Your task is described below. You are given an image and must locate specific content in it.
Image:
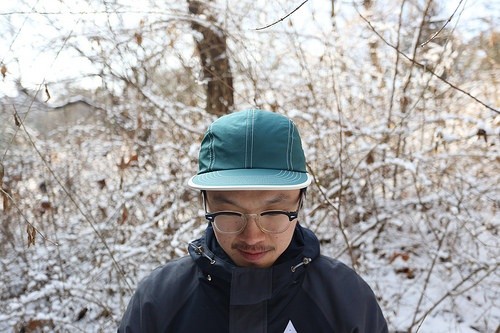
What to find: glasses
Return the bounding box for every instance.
[201,191,304,235]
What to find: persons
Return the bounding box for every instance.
[117,108,389,333]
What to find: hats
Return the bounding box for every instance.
[188,110,311,190]
